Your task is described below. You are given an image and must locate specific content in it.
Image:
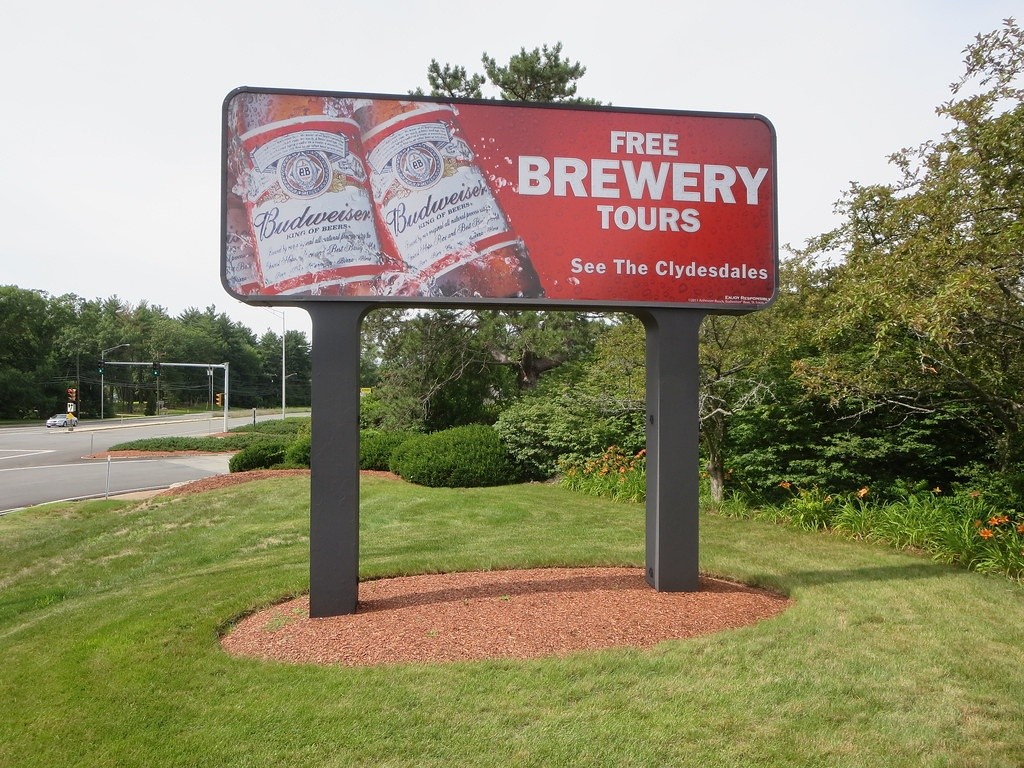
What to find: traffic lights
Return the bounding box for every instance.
[216,393,223,407]
[72,389,77,402]
[68,389,73,401]
[97,360,103,374]
[153,361,160,376]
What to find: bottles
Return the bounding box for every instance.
[351,98,544,299]
[232,92,385,296]
[226,173,262,297]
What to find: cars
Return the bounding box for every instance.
[45,414,78,427]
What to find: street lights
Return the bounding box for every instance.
[101,343,130,419]
[238,300,298,420]
